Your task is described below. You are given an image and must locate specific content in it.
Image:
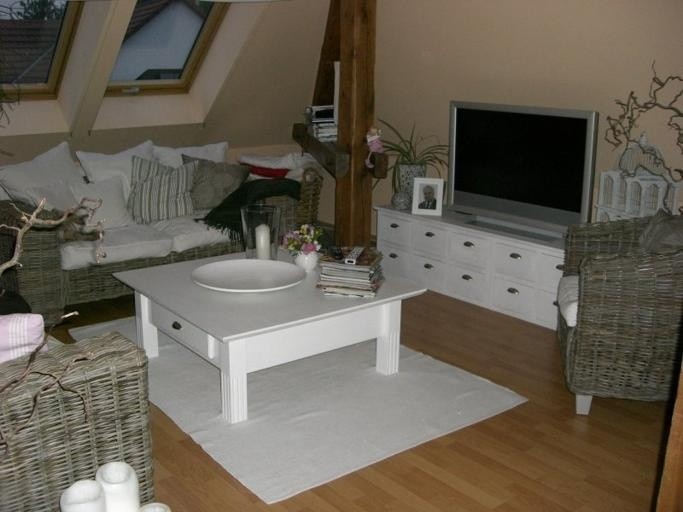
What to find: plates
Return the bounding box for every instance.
[191,258,308,294]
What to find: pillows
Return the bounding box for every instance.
[0,140,75,211]
[181,153,250,210]
[25,177,78,211]
[638,208,683,248]
[68,175,133,228]
[126,156,199,225]
[1,313,48,364]
[75,139,155,204]
[154,141,227,169]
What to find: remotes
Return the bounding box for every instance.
[329,244,343,260]
[345,246,366,265]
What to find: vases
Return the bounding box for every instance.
[391,192,411,209]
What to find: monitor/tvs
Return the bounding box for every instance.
[447,101,600,238]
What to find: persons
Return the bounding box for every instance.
[419,185,435,211]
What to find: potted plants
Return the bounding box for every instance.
[371,118,449,203]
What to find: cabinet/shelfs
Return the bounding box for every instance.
[373,204,565,332]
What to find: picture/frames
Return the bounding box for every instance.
[411,176,444,217]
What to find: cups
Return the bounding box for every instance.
[239,204,282,260]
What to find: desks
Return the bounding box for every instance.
[111,247,427,424]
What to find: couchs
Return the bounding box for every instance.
[0,143,324,327]
[556,216,683,415]
[0,290,155,511]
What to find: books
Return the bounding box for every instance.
[303,104,340,143]
[315,245,386,298]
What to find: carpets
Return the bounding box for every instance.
[68,315,528,506]
